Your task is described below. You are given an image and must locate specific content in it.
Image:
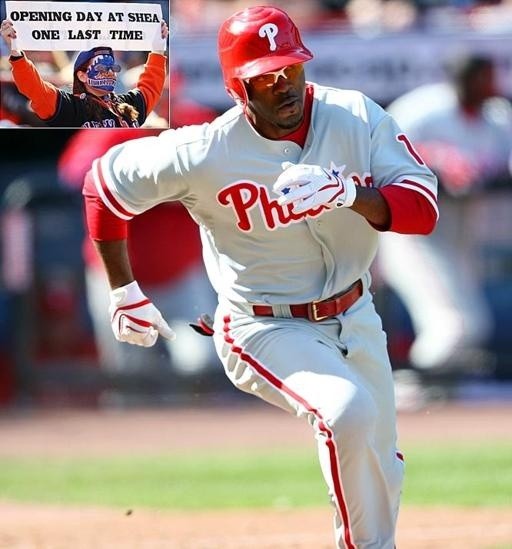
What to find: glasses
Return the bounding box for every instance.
[80,64,122,73]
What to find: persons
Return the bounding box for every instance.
[0,19,169,129]
[53,50,239,382]
[372,49,510,416]
[78,4,443,549]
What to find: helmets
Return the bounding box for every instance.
[217,14,313,109]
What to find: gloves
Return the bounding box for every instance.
[109,280,177,348]
[273,161,356,215]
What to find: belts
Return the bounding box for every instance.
[253,280,362,322]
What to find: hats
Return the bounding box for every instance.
[74,46,114,72]
